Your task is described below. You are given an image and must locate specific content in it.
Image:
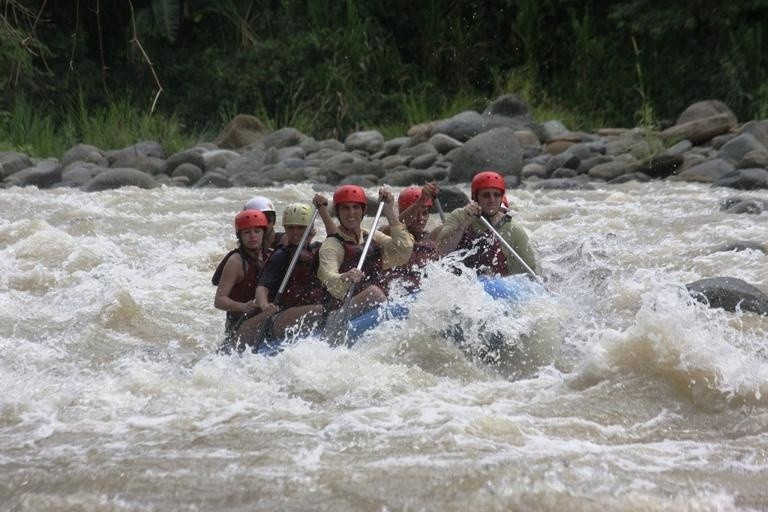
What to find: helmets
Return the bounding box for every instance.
[398,187,433,209]
[333,185,368,211]
[244,197,276,212]
[282,202,312,226]
[471,171,505,194]
[235,209,268,235]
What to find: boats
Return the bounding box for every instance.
[255,275,550,362]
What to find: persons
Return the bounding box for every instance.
[376,185,450,294]
[256,193,340,339]
[500,194,509,214]
[436,171,547,285]
[245,197,285,252]
[213,207,280,346]
[316,184,416,329]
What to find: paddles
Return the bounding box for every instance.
[249,203,328,351]
[321,199,385,346]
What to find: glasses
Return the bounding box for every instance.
[265,212,274,222]
[479,191,503,198]
